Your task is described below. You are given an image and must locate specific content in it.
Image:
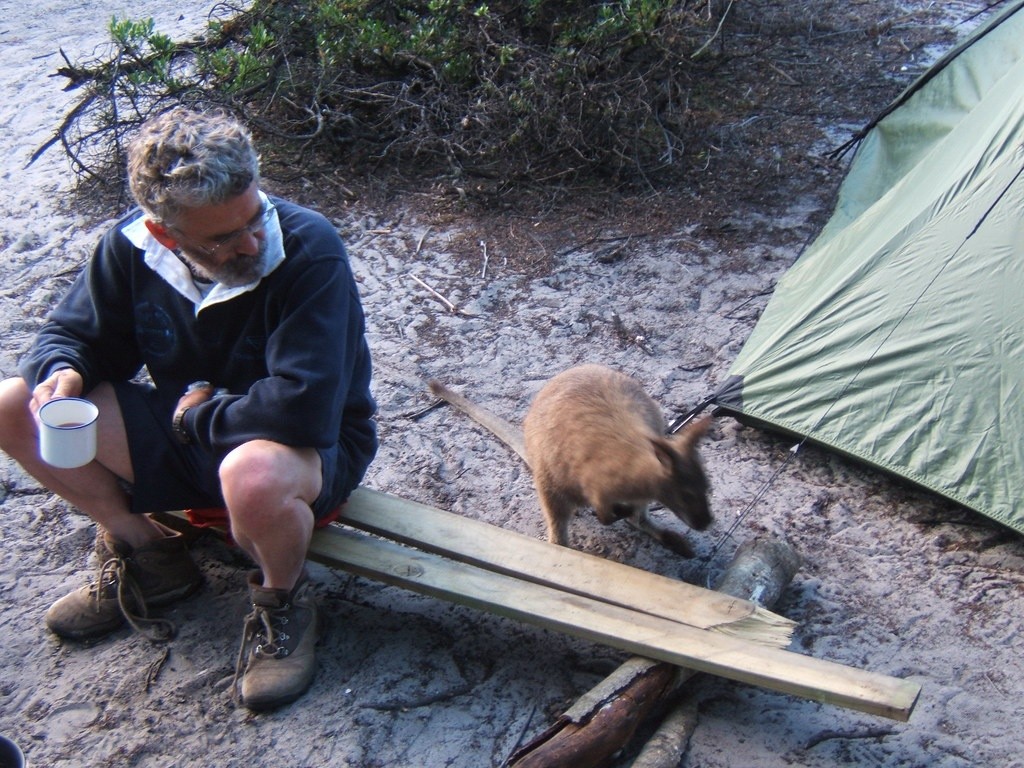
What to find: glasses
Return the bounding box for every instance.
[162,183,279,259]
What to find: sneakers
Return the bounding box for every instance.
[47,516,206,644]
[230,567,319,713]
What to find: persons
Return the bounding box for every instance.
[1,107,378,710]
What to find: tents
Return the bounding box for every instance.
[669,0,1024,533]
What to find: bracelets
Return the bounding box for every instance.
[174,407,194,446]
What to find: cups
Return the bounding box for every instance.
[38,397,99,469]
